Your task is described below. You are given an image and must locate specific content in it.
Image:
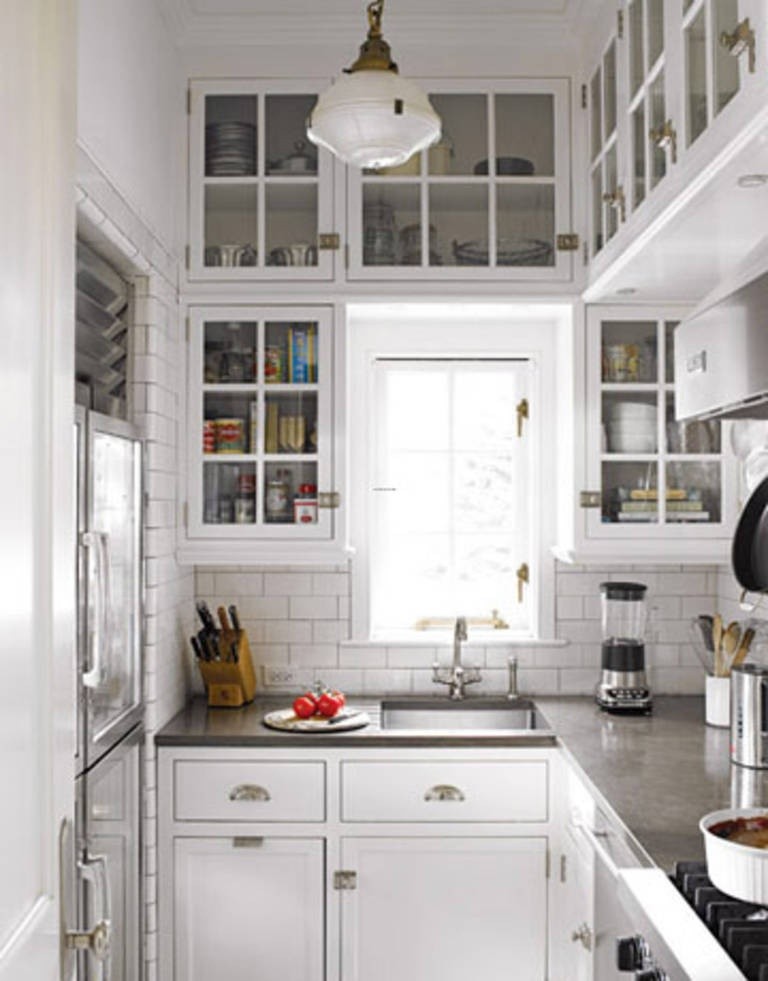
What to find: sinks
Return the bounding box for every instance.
[376,697,555,733]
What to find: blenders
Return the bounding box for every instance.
[594,583,654,713]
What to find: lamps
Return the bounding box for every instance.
[305,0,441,167]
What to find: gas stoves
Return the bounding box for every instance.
[616,859,768,980]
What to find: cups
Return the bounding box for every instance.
[600,343,639,380]
[363,203,396,263]
[731,663,768,769]
[402,224,443,265]
[704,674,730,730]
[206,120,256,175]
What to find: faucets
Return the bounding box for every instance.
[432,616,483,701]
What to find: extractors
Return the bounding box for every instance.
[673,257,768,424]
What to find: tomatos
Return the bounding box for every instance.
[294,687,346,718]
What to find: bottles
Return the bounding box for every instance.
[207,469,318,524]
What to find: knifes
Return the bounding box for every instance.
[188,600,241,663]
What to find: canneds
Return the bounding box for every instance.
[216,472,289,523]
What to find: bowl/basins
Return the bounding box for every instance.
[699,807,768,909]
[205,244,256,267]
[600,401,658,455]
[270,245,318,265]
[731,474,768,614]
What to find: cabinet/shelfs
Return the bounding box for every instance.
[170,0,768,566]
[159,744,595,981]
